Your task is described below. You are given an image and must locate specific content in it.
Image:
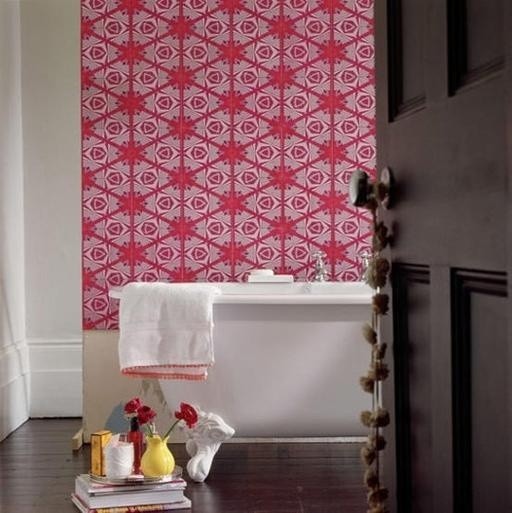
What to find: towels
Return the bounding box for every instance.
[118,283,213,381]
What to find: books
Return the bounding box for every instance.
[70,474,192,513]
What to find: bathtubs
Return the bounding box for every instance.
[105,281,379,438]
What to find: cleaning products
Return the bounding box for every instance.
[128,417,143,475]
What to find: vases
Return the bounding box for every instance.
[140,434,175,477]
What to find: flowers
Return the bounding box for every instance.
[124,396,158,437]
[162,400,198,442]
